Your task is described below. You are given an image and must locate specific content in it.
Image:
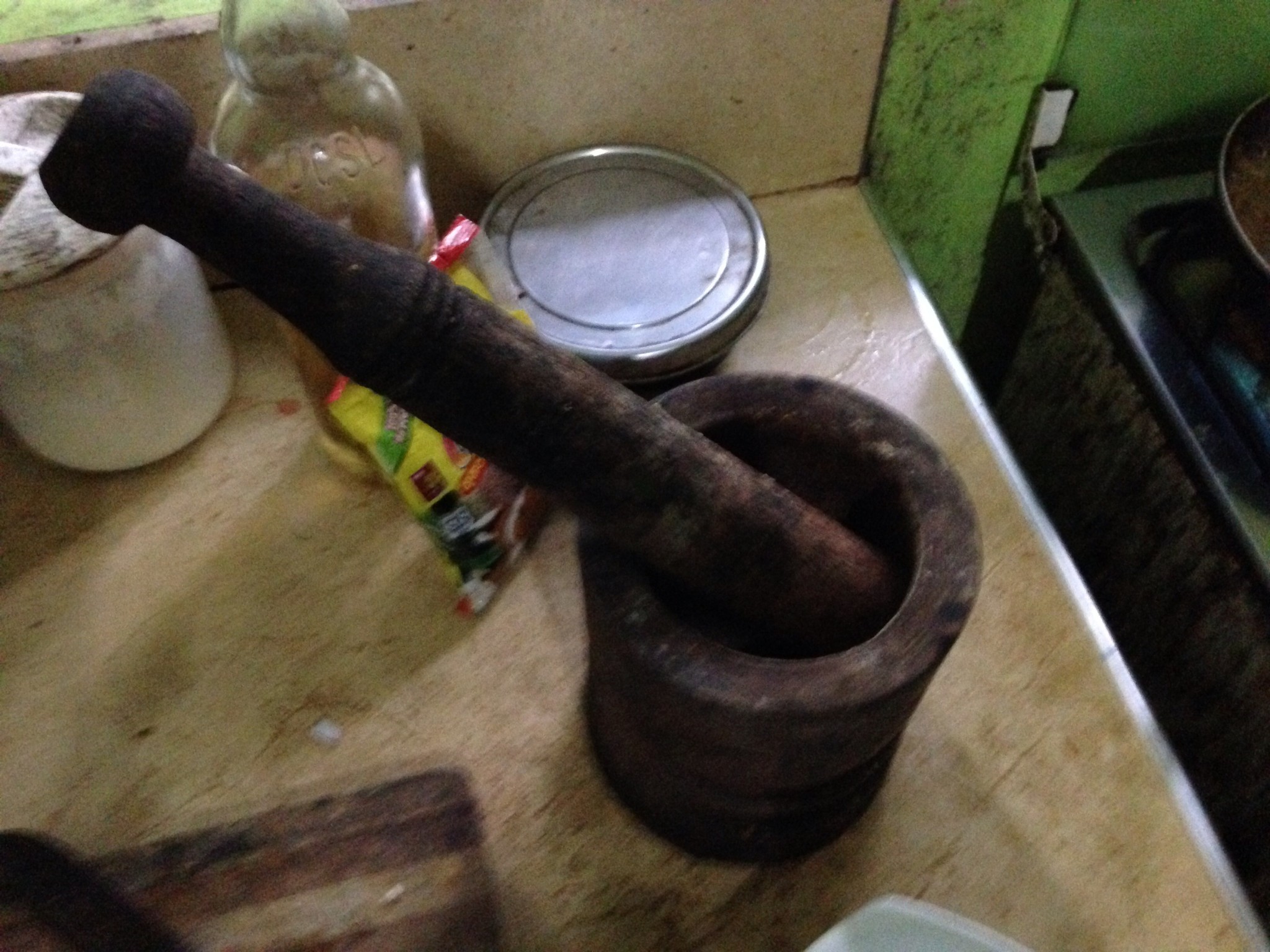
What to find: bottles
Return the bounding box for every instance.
[211,0,442,483]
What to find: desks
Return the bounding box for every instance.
[2,173,1268,952]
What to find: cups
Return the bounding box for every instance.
[585,367,984,872]
